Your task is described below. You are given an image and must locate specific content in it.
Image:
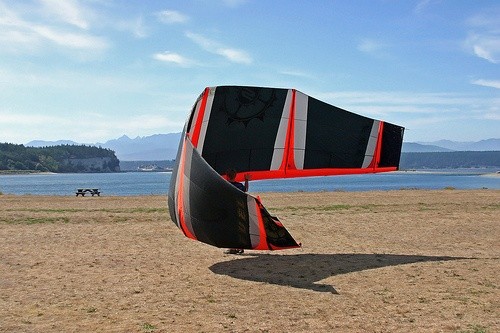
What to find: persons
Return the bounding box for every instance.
[224,169,251,255]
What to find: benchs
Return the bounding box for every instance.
[75,192,100,197]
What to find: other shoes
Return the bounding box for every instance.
[224,249,244,255]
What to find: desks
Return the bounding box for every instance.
[75,187,101,197]
